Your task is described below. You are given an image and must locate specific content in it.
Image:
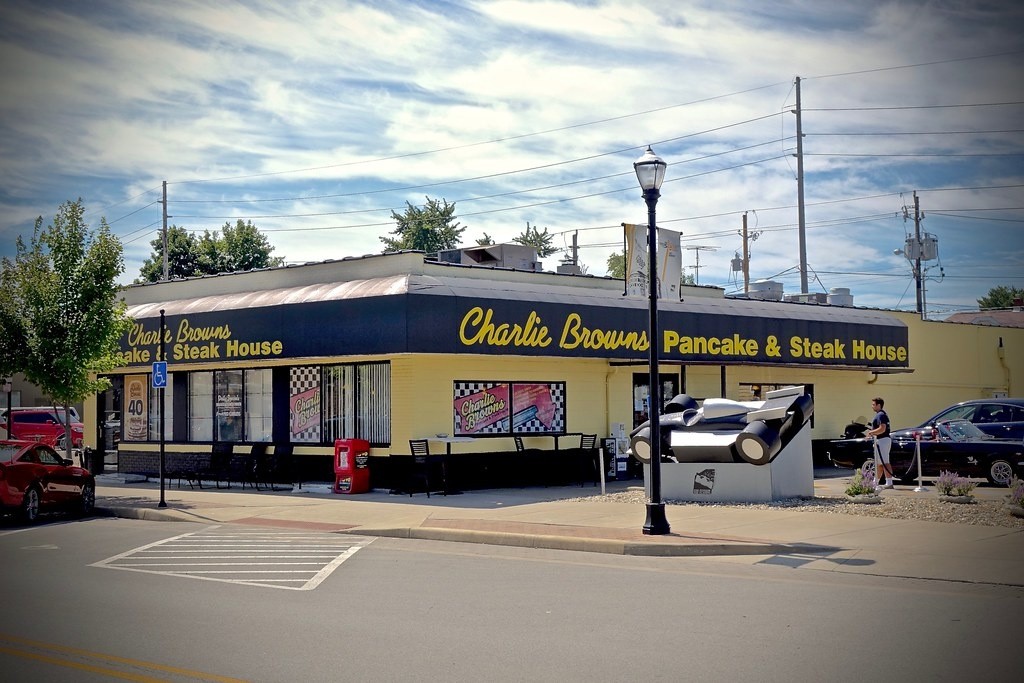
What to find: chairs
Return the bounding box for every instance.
[168,440,296,490]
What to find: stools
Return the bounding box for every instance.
[408,439,447,499]
[574,434,597,488]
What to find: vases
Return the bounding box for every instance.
[939,494,973,504]
[1009,506,1024,516]
[844,494,882,504]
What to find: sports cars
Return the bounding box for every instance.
[826,418,1024,488]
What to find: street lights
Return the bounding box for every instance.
[894,249,923,314]
[632,144,673,536]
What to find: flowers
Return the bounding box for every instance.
[933,469,974,496]
[1004,475,1024,506]
[846,466,877,495]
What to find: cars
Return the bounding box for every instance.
[0,439,96,526]
[909,397,1024,444]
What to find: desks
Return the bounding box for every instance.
[422,437,475,495]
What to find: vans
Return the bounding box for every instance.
[6,409,84,451]
[0,406,81,440]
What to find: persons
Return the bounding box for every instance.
[866,398,894,489]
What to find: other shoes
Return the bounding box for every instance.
[883,484,893,489]
